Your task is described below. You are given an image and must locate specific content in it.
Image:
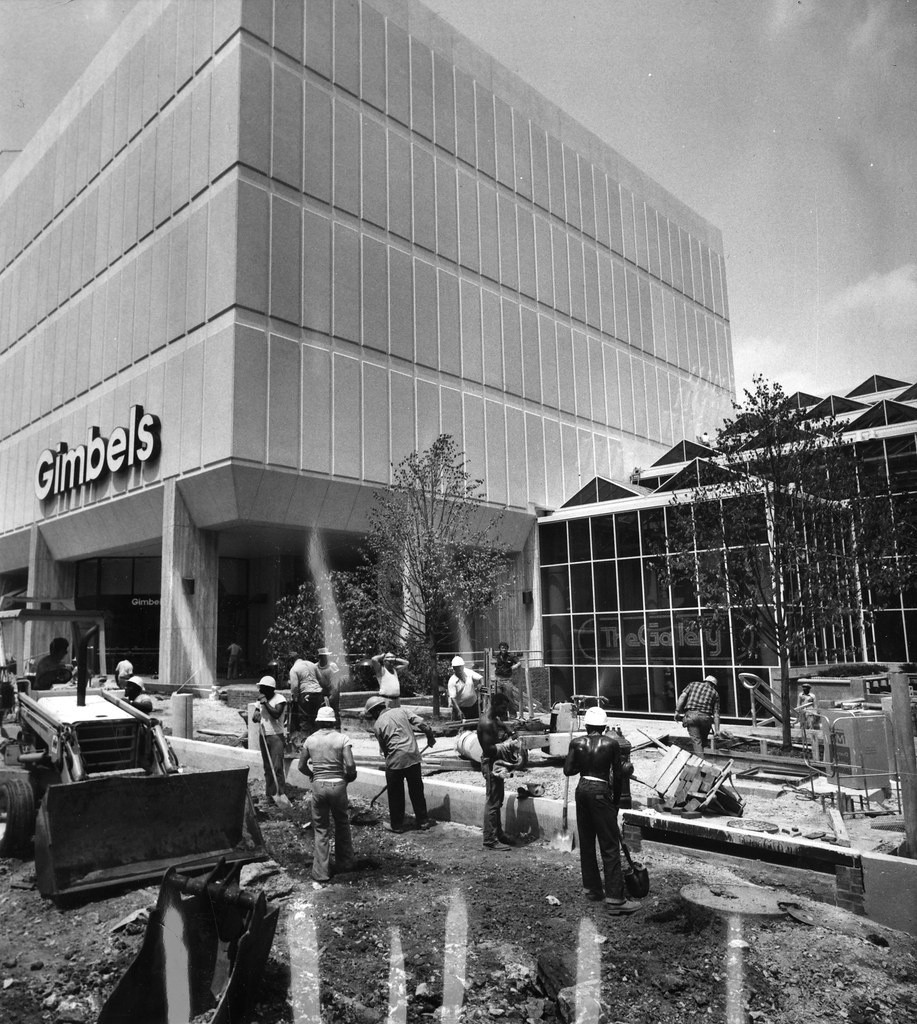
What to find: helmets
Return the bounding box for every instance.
[125,676,145,691]
[364,696,386,717]
[452,656,465,666]
[256,676,276,688]
[584,707,608,726]
[314,706,337,722]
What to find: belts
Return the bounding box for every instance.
[381,695,400,699]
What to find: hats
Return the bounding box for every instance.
[802,684,812,690]
[704,675,718,685]
[317,648,333,655]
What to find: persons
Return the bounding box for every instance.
[121,676,153,713]
[115,653,134,689]
[563,706,643,916]
[297,706,358,888]
[314,647,341,729]
[226,640,242,680]
[371,651,410,756]
[447,655,484,730]
[477,692,518,851]
[495,641,522,719]
[252,675,287,804]
[288,650,329,732]
[33,637,72,690]
[365,694,436,834]
[797,683,818,729]
[674,674,721,760]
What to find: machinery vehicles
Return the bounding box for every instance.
[0,594,272,900]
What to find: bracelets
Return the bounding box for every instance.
[675,709,680,712]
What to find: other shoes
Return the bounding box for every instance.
[498,836,516,846]
[483,840,511,851]
[607,901,642,914]
[383,820,403,833]
[585,889,605,900]
[350,857,368,872]
[418,818,436,829]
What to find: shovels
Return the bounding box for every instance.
[257,718,293,809]
[615,816,650,900]
[366,744,428,810]
[548,720,574,854]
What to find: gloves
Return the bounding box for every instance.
[257,693,268,704]
[251,711,260,723]
